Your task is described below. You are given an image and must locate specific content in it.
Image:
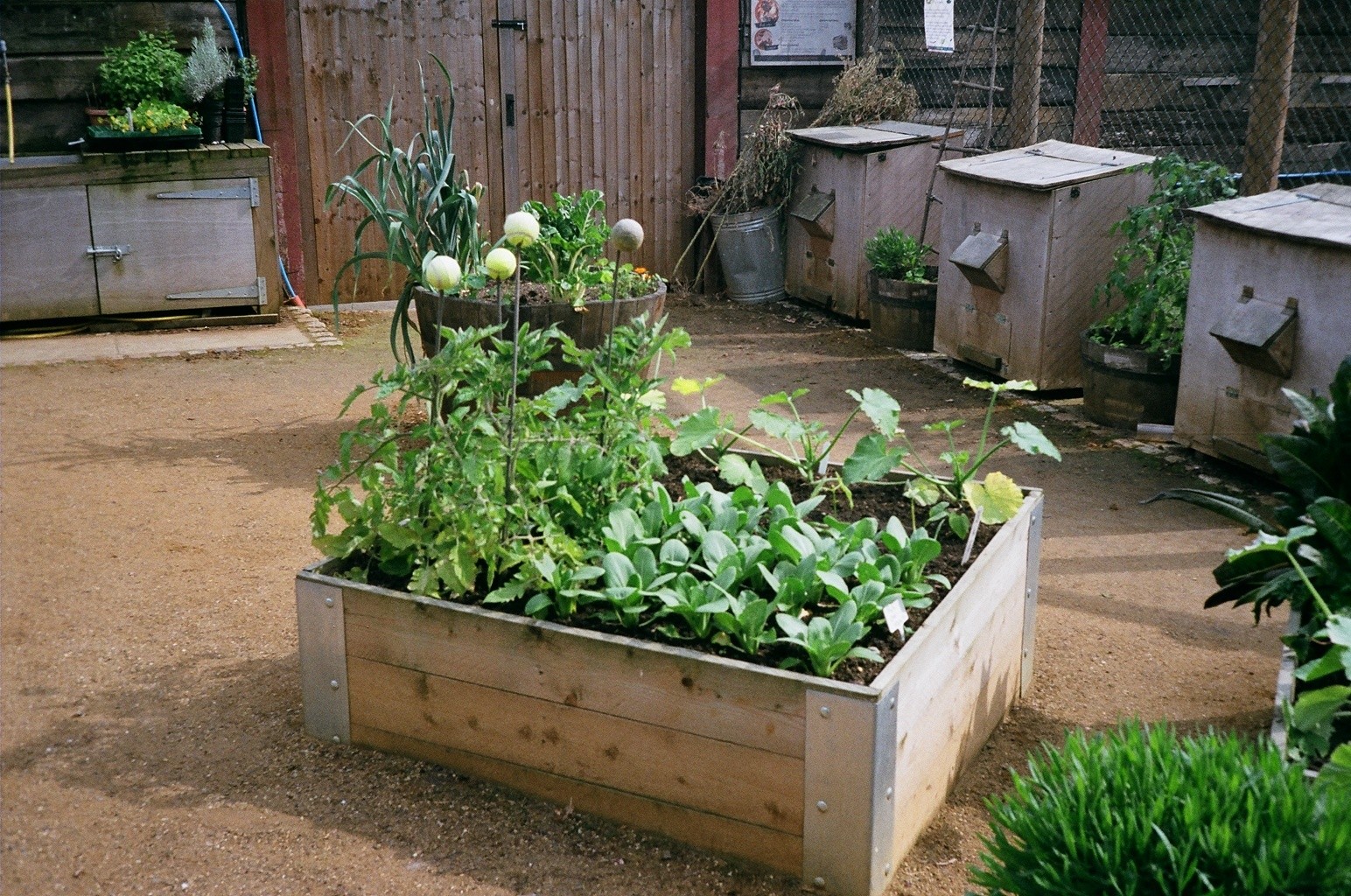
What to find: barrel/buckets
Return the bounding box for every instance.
[710,202,787,305]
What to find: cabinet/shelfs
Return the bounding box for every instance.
[0,138,282,336]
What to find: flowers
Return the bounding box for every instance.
[179,15,262,105]
[96,93,204,134]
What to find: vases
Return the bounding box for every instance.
[197,97,225,145]
[83,105,126,125]
[86,126,205,153]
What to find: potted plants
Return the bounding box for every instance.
[1078,152,1243,432]
[320,48,666,441]
[294,211,1064,896]
[861,222,938,353]
[1138,350,1351,782]
